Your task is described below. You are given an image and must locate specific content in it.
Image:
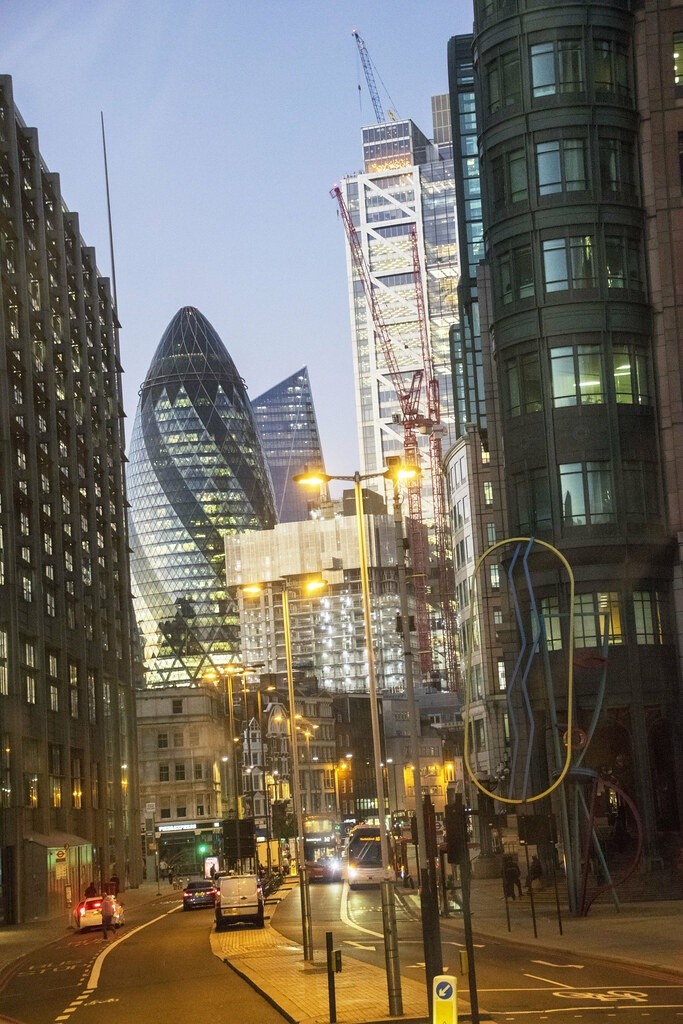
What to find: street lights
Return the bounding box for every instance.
[256,684,276,882]
[237,578,329,968]
[201,668,257,876]
[292,460,420,1015]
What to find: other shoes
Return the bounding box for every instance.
[113,930,116,935]
[103,937,108,939]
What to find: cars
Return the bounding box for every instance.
[182,879,217,911]
[73,894,126,934]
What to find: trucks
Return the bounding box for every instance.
[214,874,264,931]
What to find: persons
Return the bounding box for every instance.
[101,892,118,940]
[524,856,541,888]
[84,882,96,898]
[210,864,217,878]
[504,857,525,901]
[110,874,120,900]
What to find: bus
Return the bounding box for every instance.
[303,831,342,884]
[344,826,399,889]
